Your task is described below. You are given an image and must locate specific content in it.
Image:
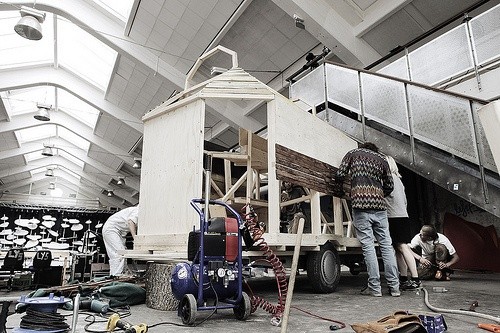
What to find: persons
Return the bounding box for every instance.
[407,225,460,281]
[102,207,141,277]
[337,142,423,297]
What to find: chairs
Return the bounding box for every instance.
[0,248,105,289]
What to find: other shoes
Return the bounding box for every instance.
[391,290,402,296]
[400,279,423,289]
[276,246,286,251]
[361,287,382,296]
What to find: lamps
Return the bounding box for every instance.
[34,103,142,197]
[14,0,47,41]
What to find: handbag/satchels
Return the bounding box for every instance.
[91,279,146,307]
[418,313,448,333]
[350,310,428,333]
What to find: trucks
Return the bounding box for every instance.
[120,45,409,294]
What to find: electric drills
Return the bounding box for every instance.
[107,313,150,333]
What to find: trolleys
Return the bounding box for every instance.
[170,199,252,326]
